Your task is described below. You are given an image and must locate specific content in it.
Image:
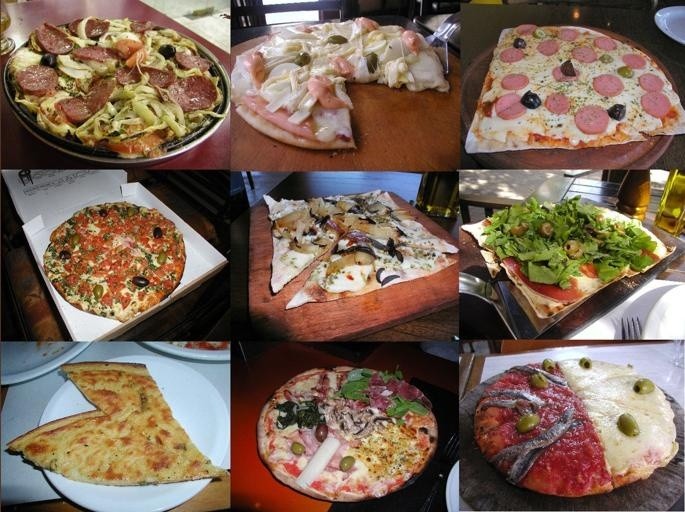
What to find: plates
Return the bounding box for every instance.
[1,341,231,512]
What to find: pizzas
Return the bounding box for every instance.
[260,189,458,310]
[465,22,685,154]
[43,203,187,323]
[256,366,439,503]
[5,361,226,487]
[231,15,450,150]
[463,199,676,320]
[473,357,680,498]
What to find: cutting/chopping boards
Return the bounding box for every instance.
[230,24,460,172]
[459,357,684,512]
[248,190,458,342]
[460,24,678,170]
